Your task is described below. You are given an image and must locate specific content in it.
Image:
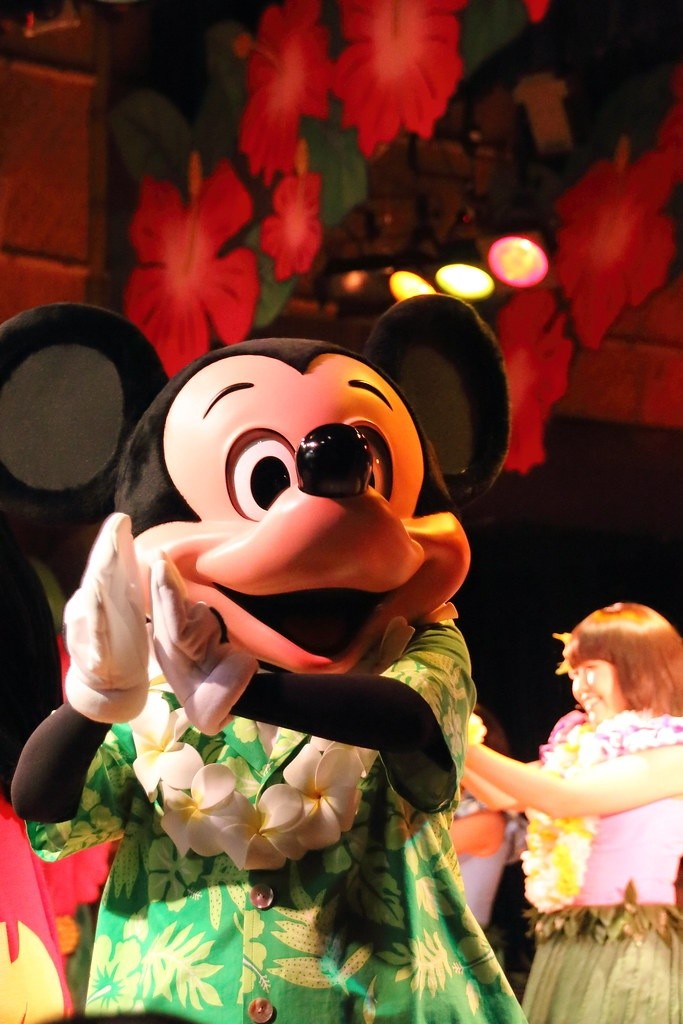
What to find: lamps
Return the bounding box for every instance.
[390,201,563,313]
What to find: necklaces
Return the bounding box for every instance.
[519,711,683,915]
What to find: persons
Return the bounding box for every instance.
[454,603,683,1024]
[455,707,515,931]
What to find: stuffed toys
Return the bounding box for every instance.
[0,287,526,1024]
